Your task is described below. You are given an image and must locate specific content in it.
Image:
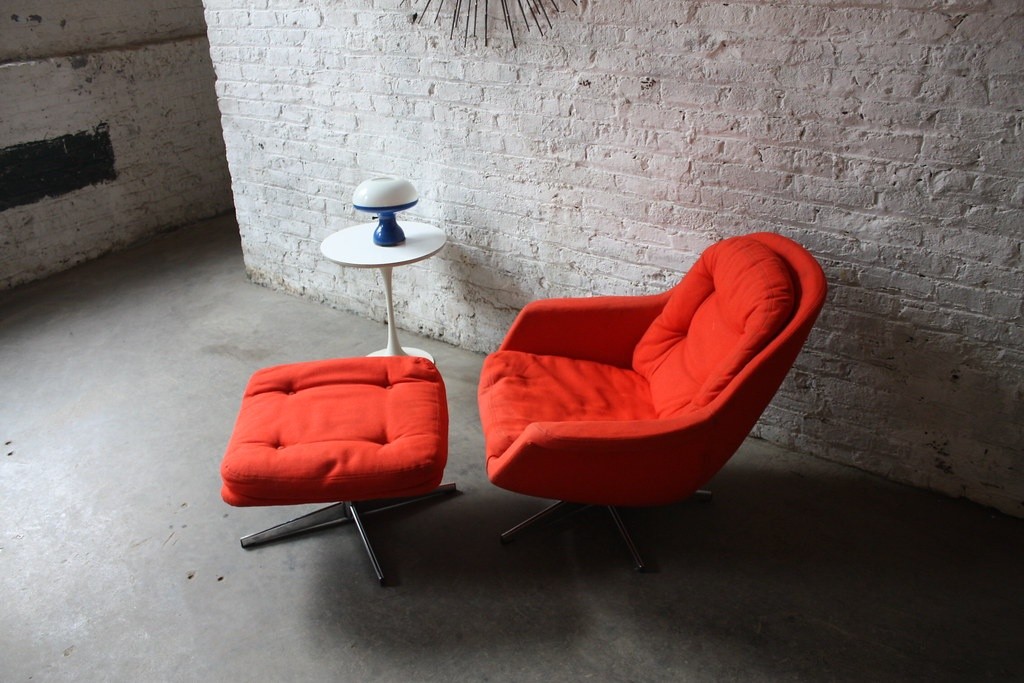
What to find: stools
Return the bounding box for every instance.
[221,357,456,586]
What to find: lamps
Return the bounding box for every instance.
[352,177,419,247]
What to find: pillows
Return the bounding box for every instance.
[632,235,796,418]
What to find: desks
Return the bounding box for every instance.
[321,220,448,365]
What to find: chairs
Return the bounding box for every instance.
[475,231,829,570]
